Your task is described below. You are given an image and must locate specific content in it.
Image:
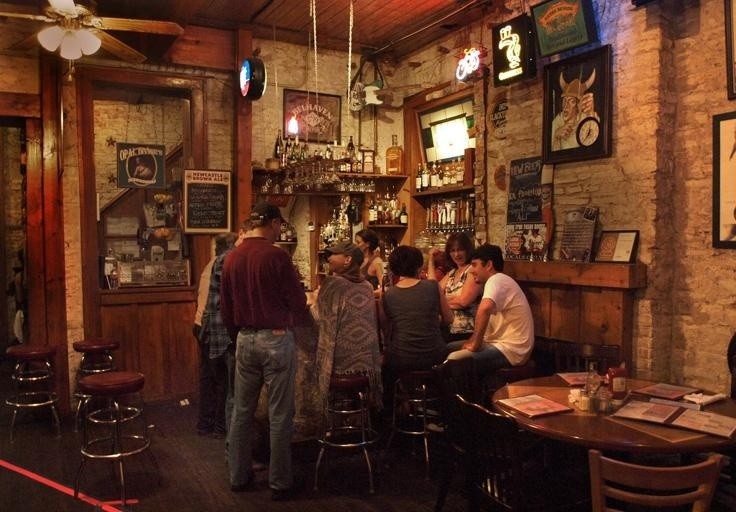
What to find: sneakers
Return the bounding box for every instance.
[426,422,449,433]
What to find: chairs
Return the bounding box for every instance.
[587,449,725,512]
[547,341,621,374]
[434,392,590,512]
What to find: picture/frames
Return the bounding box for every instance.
[592,230,639,263]
[712,112,736,249]
[541,45,612,165]
[530,0,598,59]
[103,214,141,238]
[116,142,166,189]
[283,88,342,145]
[723,0,736,101]
[104,238,143,260]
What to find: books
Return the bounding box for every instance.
[557,372,609,385]
[633,381,703,399]
[613,399,736,439]
[495,394,573,416]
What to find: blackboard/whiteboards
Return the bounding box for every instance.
[504,182,554,263]
[506,155,544,225]
[560,206,598,263]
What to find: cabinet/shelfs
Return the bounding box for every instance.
[252,166,408,292]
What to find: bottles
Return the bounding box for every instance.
[579,361,613,412]
[415,157,464,193]
[377,234,400,262]
[280,225,297,242]
[274,129,362,173]
[386,135,405,174]
[368,183,409,227]
[353,205,361,225]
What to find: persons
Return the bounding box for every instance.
[445,244,534,392]
[132,156,153,179]
[426,232,480,337]
[297,241,380,424]
[193,220,253,439]
[552,69,600,151]
[355,230,383,291]
[222,202,306,501]
[378,244,451,423]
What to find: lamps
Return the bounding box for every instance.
[33,20,103,60]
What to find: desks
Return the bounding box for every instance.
[491,372,735,456]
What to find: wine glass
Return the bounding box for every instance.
[262,163,377,195]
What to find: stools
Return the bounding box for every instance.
[72,338,120,434]
[312,374,380,497]
[382,370,438,481]
[490,366,532,381]
[5,345,62,442]
[74,371,158,508]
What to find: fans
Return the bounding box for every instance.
[0,0,184,64]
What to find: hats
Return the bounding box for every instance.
[325,242,365,265]
[248,201,289,224]
